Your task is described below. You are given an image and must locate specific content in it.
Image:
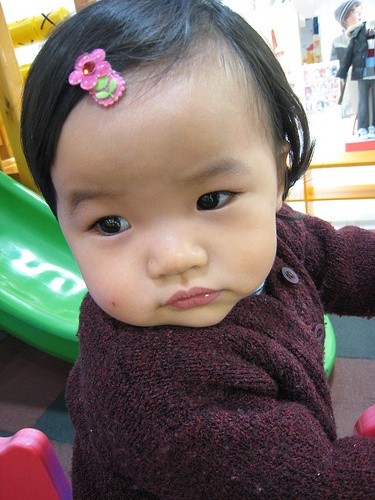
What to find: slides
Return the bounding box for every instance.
[1,172,336,382]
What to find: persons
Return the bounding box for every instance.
[334,19,375,136]
[329,1,364,63]
[21,0,375,499]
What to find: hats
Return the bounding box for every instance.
[334,1,359,27]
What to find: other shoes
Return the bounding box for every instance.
[358,126,375,139]
